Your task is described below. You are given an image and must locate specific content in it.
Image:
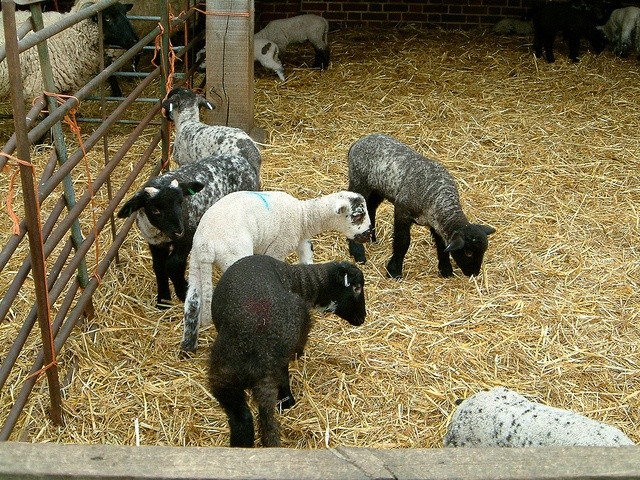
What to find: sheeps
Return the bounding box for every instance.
[117,154,261,322]
[597,6,640,61]
[162,88,262,190]
[443,385,636,448]
[179,190,376,360]
[491,18,536,35]
[1,0,141,148]
[71,1,208,98]
[254,14,331,76]
[207,254,368,447]
[347,133,498,281]
[521,5,609,63]
[196,40,287,82]
